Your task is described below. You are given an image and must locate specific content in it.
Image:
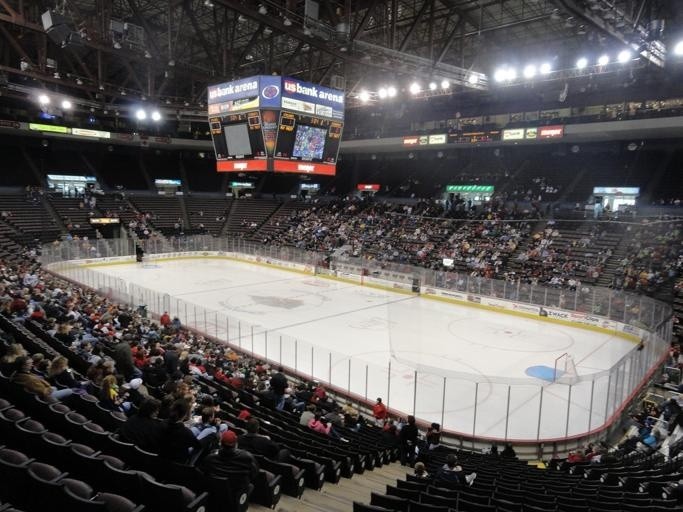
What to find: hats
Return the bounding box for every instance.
[130,378,143,389]
[222,431,236,443]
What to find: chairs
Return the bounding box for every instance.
[356,443,681,505]
[2,317,91,372]
[2,382,421,509]
